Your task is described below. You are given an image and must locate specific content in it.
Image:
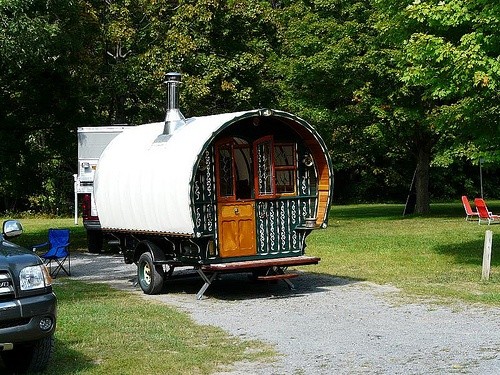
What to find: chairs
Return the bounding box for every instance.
[33,228,72,279]
[461,196,493,222]
[474,198,500,226]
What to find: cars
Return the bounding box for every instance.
[0,219,59,375]
